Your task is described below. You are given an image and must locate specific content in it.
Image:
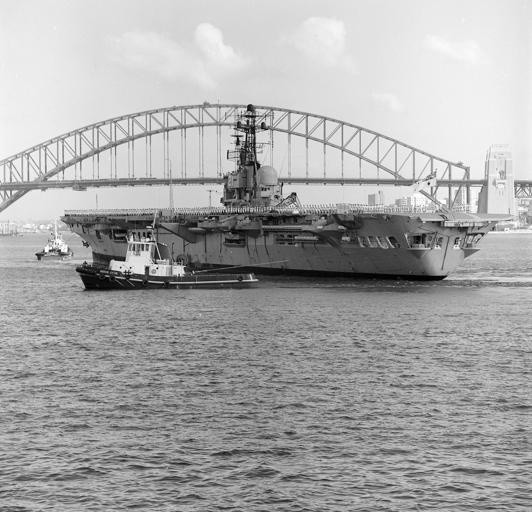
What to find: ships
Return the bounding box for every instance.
[61,103,513,281]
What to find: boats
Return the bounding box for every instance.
[75,211,289,290]
[35,222,74,262]
